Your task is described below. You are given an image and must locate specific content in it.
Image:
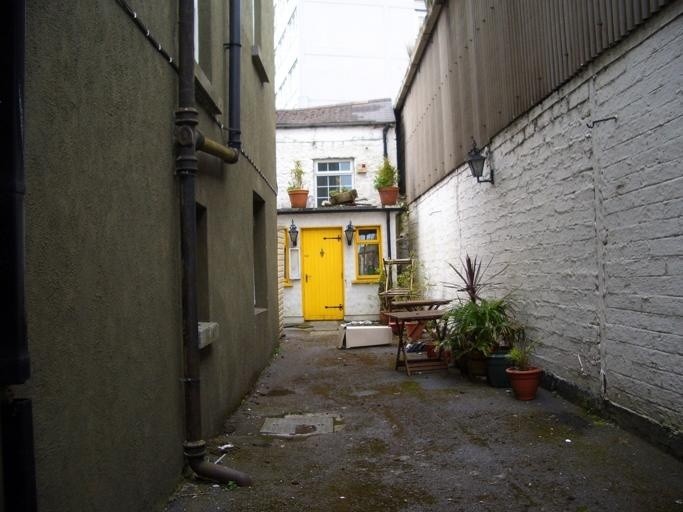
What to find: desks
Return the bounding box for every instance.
[385,294,453,378]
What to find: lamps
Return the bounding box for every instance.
[468,137,495,185]
[288,218,299,246]
[345,220,355,245]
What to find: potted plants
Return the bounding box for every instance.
[287,159,311,208]
[375,157,400,205]
[428,259,527,388]
[505,344,544,401]
